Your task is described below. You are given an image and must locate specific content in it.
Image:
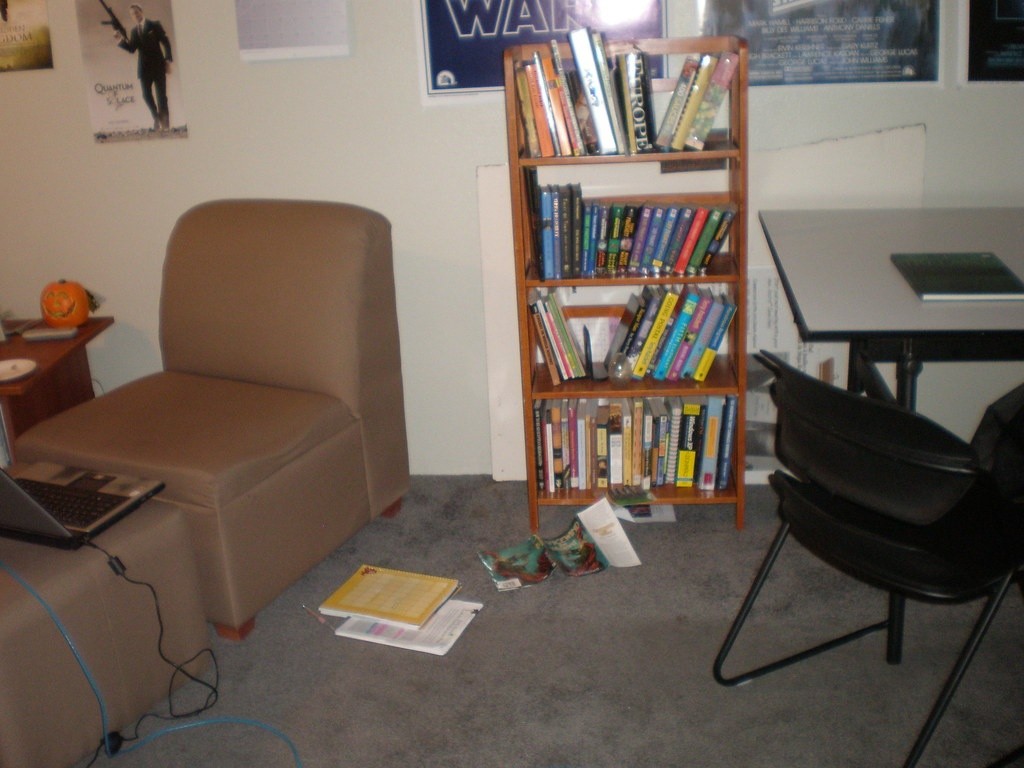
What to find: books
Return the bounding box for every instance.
[890,251,1024,302]
[319,563,484,658]
[531,393,738,498]
[527,287,587,386]
[514,26,740,159]
[603,284,738,383]
[522,165,737,283]
[477,498,643,593]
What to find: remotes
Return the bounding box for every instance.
[22,326,77,341]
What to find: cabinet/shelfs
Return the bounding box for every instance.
[502,35,749,538]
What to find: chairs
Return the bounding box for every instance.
[711,341,1024,768]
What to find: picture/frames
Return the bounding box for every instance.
[959,0,1024,86]
[413,0,677,105]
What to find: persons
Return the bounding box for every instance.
[112,4,173,132]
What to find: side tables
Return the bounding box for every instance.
[0,318,114,463]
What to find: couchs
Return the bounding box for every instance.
[0,497,212,768]
[15,196,411,643]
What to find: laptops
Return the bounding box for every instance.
[0,462,165,550]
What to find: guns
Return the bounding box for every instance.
[98,0,130,44]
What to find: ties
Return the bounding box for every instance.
[138,23,143,37]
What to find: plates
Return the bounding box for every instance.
[0,359,36,383]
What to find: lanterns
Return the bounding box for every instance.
[39,279,89,329]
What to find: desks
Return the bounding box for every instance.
[757,206,1024,693]
